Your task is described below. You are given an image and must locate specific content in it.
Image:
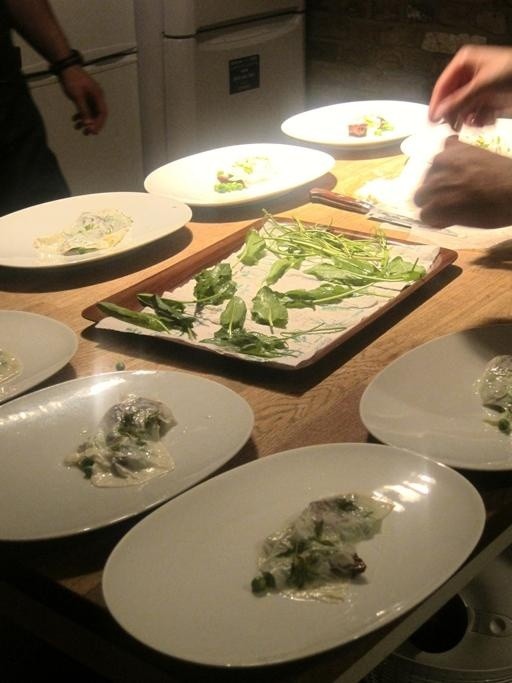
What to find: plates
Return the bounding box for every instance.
[0,191,194,273]
[357,321,511,474]
[279,99,445,151]
[142,142,335,209]
[399,117,512,160]
[79,213,460,376]
[99,440,489,667]
[1,307,257,544]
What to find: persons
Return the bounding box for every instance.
[0,0,108,216]
[413,43,512,230]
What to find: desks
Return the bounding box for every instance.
[0,112,512,683]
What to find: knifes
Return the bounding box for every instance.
[306,185,461,239]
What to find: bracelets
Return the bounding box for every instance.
[48,49,84,76]
